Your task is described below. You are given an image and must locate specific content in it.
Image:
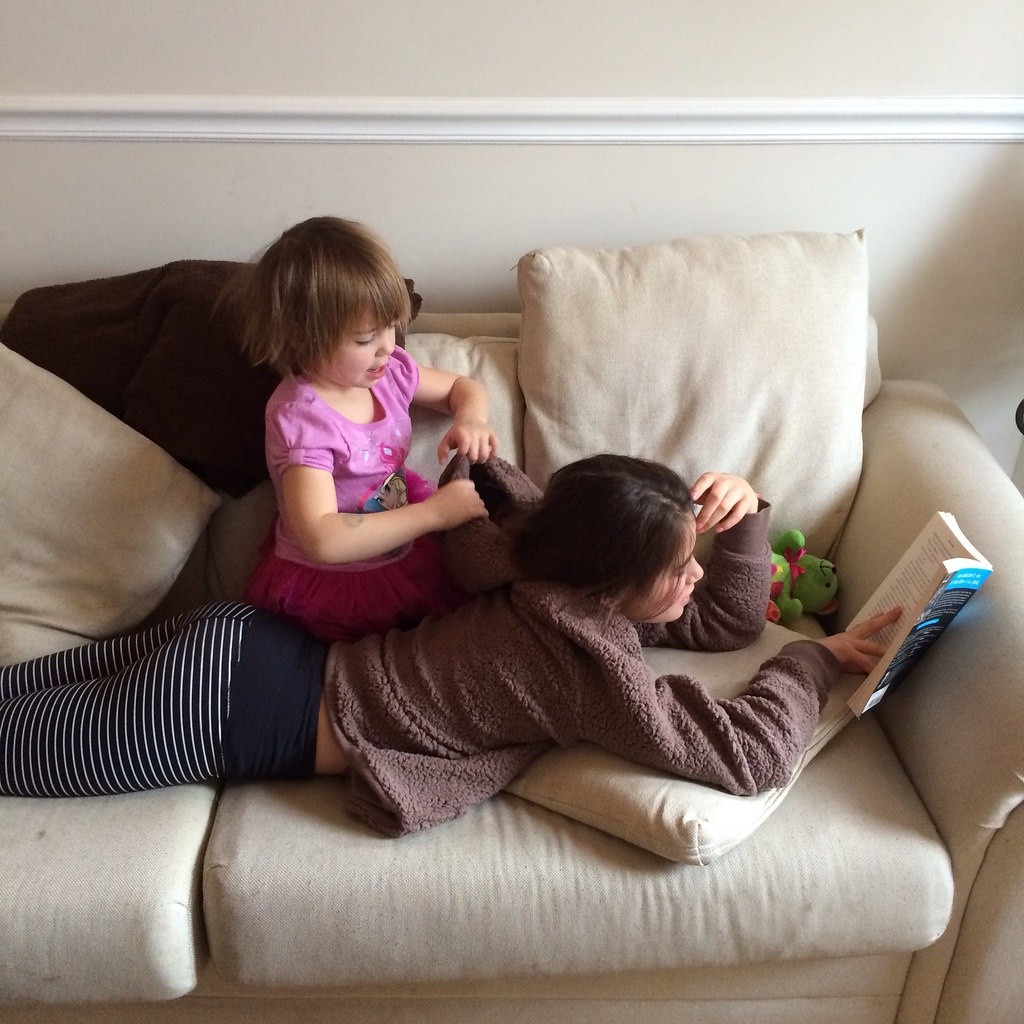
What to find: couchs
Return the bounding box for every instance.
[0,311,1023,1024]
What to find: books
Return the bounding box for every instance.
[845,510,992,723]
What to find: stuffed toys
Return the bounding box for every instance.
[767,526,842,624]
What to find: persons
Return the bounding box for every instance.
[243,216,502,644]
[2,448,904,841]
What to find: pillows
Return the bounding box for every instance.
[511,227,868,586]
[205,333,524,608]
[0,340,225,672]
[497,619,868,867]
